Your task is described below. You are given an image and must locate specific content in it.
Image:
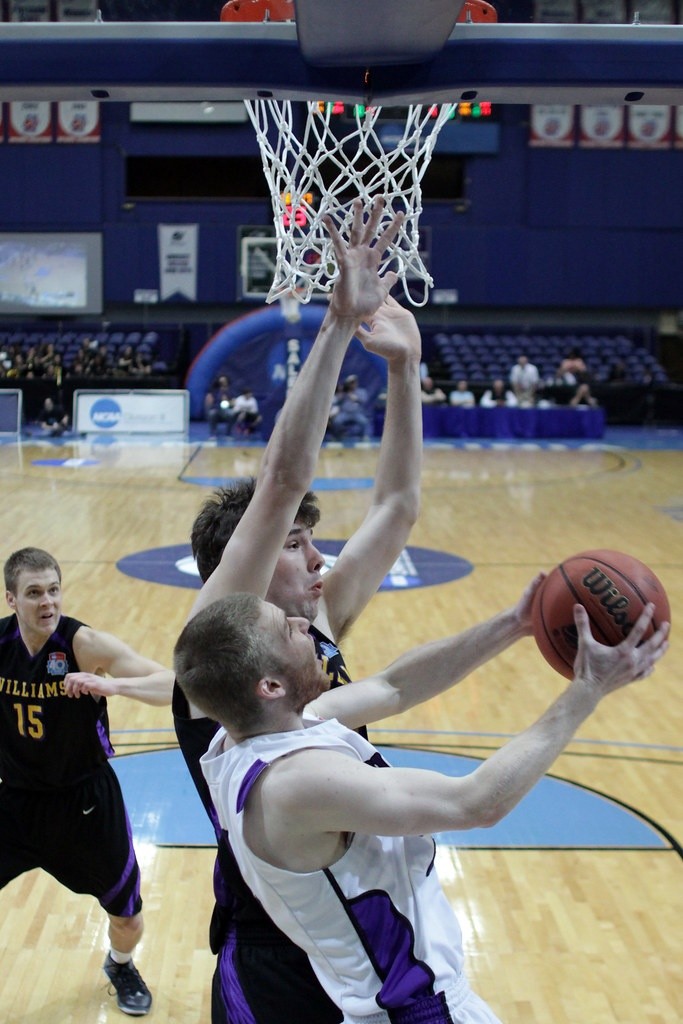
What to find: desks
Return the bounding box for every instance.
[372,407,607,441]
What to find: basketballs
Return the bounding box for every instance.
[532,547,672,684]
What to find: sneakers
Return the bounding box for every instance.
[103,950,152,1015]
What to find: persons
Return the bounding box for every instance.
[0,339,153,432]
[173,572,671,1024]
[420,352,598,410]
[0,547,175,1016]
[170,199,422,1023]
[201,374,372,443]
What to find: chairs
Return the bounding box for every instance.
[0,329,172,388]
[430,329,677,388]
